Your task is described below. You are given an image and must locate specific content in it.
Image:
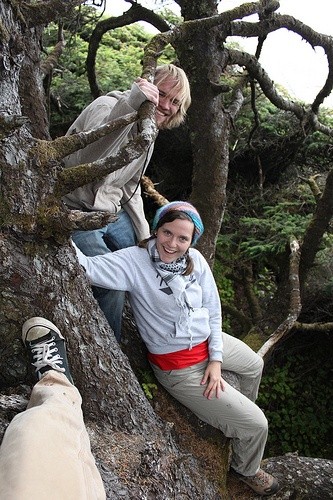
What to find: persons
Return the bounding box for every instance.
[61,64,192,342]
[0,315,108,500]
[70,200,280,495]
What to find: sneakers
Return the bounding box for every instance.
[230,468,279,496]
[22,317,74,388]
